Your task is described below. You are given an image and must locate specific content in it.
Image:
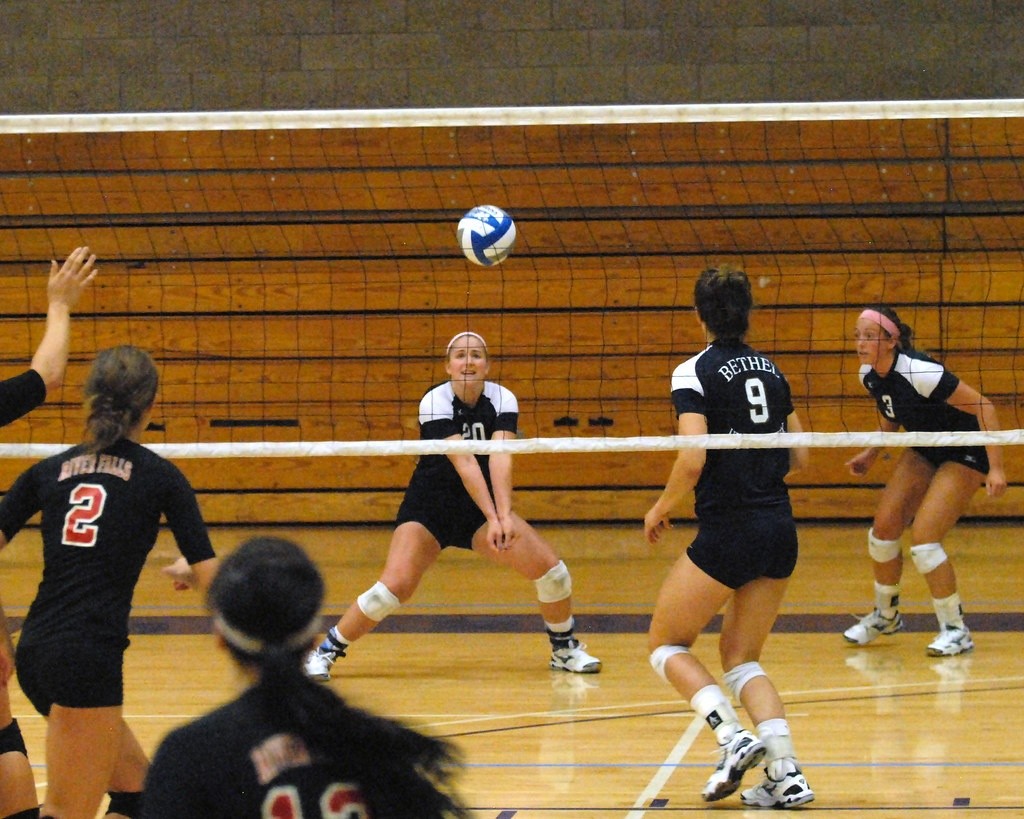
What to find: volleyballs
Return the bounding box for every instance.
[456,203,517,268]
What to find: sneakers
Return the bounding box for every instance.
[844,607,903,644]
[303,646,336,681]
[739,767,815,809]
[550,640,602,673]
[701,728,767,802]
[927,622,974,657]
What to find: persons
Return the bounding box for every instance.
[296,331,605,682]
[841,303,1008,659]
[642,264,816,809]
[136,533,473,818]
[0,238,224,819]
